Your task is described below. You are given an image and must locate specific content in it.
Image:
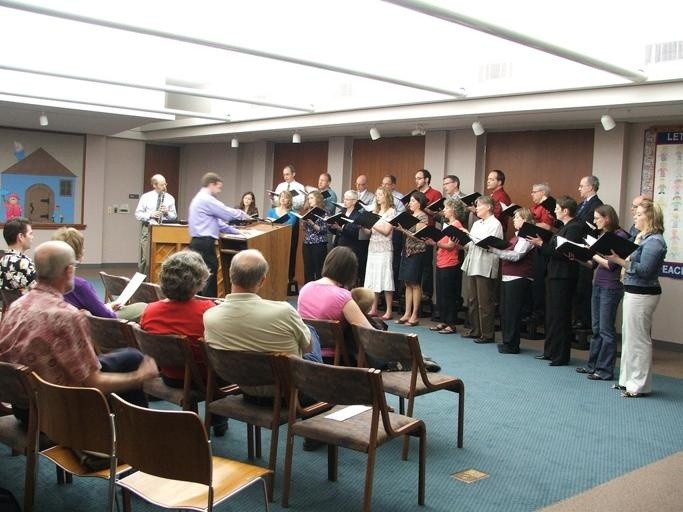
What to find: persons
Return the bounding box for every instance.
[235,192,258,218]
[266,165,511,344]
[136,173,177,276]
[0,217,160,405]
[189,172,253,299]
[141,246,389,450]
[489,176,667,395]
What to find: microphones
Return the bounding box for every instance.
[259,218,274,226]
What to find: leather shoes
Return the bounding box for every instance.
[575,367,614,380]
[570,320,585,330]
[536,354,570,366]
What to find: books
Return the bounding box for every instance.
[450,468,490,483]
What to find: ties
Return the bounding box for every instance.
[156,193,165,210]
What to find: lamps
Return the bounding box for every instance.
[472,121,485,136]
[39,111,49,126]
[369,127,382,141]
[600,115,616,133]
[292,130,302,144]
[230,135,239,148]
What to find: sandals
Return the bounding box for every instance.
[368,312,394,320]
[612,383,651,398]
[430,323,456,334]
[395,318,420,326]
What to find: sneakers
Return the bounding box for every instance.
[498,342,521,354]
[213,422,228,438]
[462,328,496,343]
[420,353,442,373]
[302,437,327,451]
